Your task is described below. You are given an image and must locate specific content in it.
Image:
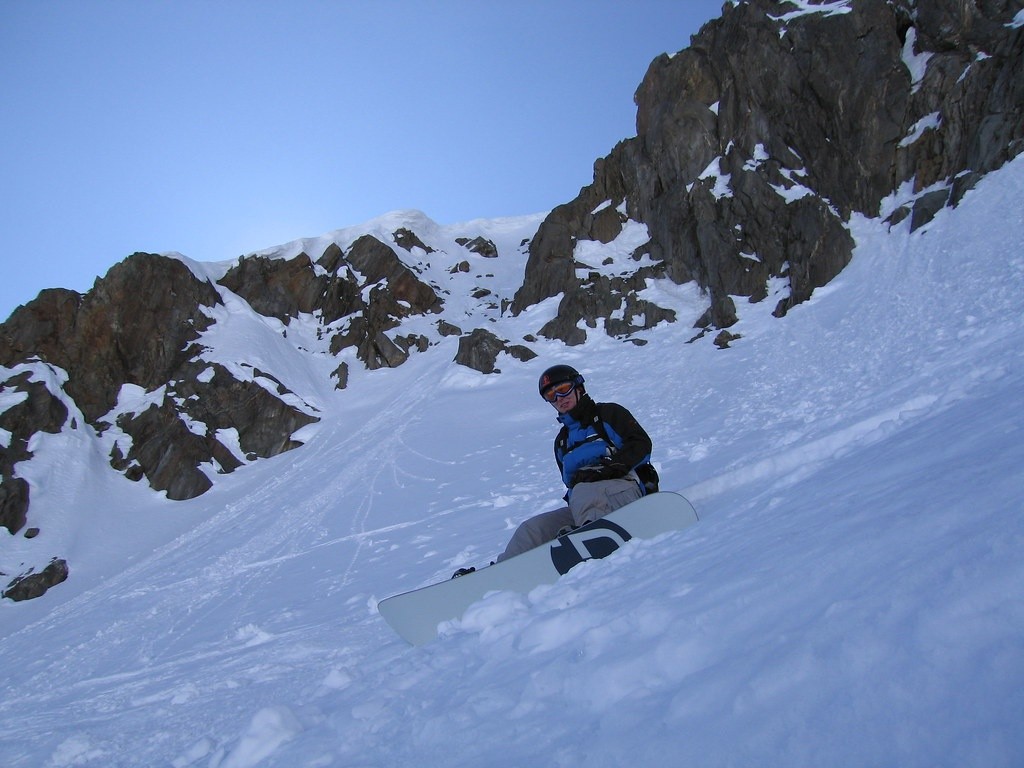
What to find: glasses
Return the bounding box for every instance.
[542,375,585,402]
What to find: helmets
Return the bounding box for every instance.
[539,365,579,394]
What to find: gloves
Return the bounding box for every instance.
[570,462,628,489]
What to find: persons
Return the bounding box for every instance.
[451,364,660,578]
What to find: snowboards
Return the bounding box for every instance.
[376,491,700,644]
